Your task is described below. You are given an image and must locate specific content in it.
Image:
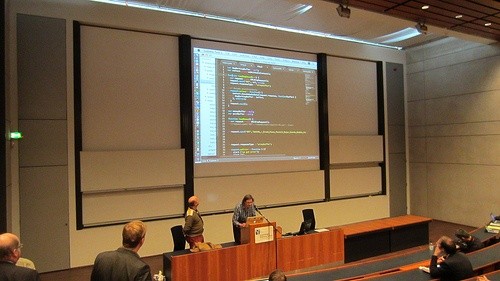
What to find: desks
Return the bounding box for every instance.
[162,241,251,281]
[259,216,500,281]
[276,215,433,275]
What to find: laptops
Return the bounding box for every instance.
[244,215,265,227]
[490,212,500,223]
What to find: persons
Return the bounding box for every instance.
[0,232,39,281]
[455,228,477,254]
[232,194,256,245]
[429,236,475,281]
[182,196,204,247]
[90,220,152,281]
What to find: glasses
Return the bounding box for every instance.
[16,244,24,249]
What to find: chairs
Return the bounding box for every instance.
[171,225,187,251]
[299,208,316,234]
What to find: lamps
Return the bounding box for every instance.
[416,18,427,34]
[336,0,351,19]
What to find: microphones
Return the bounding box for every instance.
[254,204,260,213]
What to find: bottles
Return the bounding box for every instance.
[429,239,433,251]
[253,217,257,223]
